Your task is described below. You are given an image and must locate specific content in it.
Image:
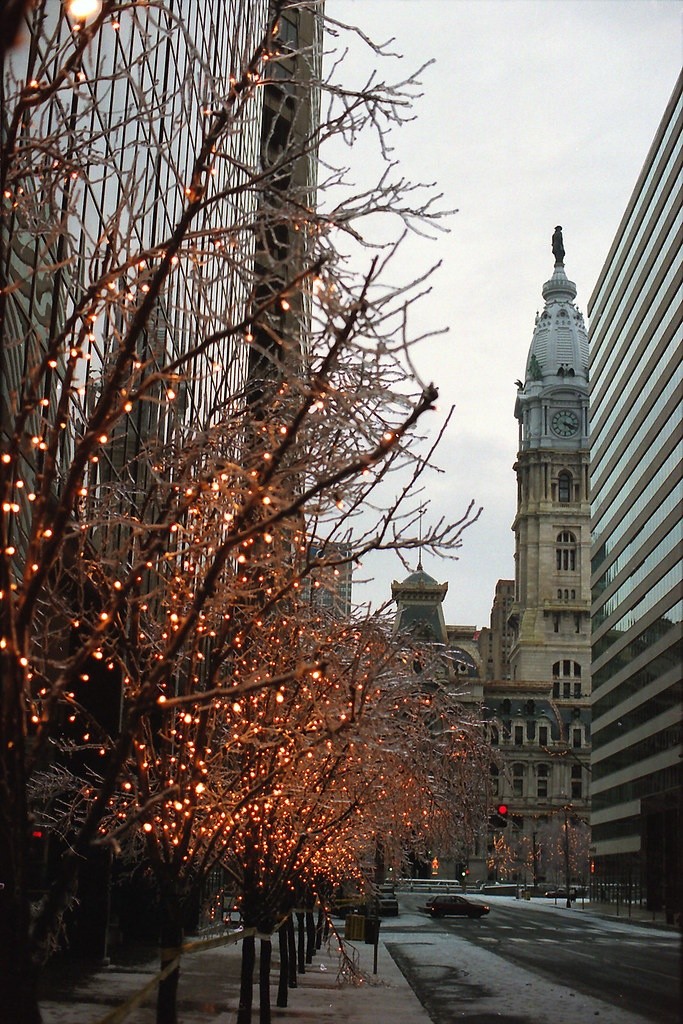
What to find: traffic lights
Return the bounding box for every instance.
[461,869,470,876]
[498,806,509,827]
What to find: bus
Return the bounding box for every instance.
[384,877,460,892]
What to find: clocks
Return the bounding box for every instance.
[550,408,580,439]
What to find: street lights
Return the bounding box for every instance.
[537,803,571,908]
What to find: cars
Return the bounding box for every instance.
[323,886,369,917]
[544,888,568,899]
[376,886,398,916]
[419,896,490,920]
